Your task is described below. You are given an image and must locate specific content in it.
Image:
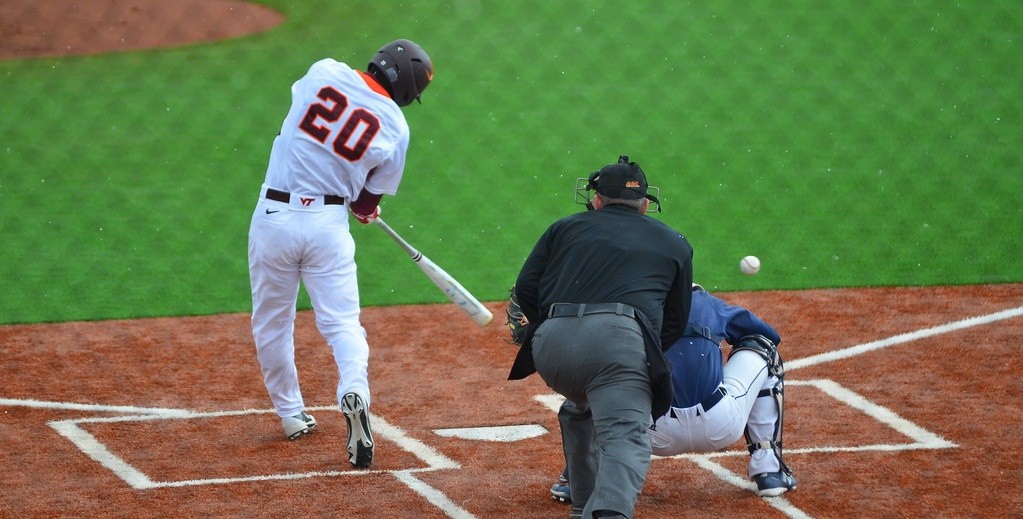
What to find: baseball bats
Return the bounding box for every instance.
[374,216,495,329]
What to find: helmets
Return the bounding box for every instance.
[369,40,434,107]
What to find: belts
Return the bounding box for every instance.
[553,303,640,320]
[669,387,727,418]
[265,188,345,206]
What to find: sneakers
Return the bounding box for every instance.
[282,411,316,440]
[756,472,798,497]
[341,392,374,468]
[551,475,572,502]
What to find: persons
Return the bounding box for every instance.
[248,39,434,469]
[550,283,797,504]
[507,156,693,519]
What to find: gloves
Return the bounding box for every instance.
[347,203,382,226]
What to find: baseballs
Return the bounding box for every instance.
[739,255,762,275]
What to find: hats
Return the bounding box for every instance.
[595,163,648,200]
